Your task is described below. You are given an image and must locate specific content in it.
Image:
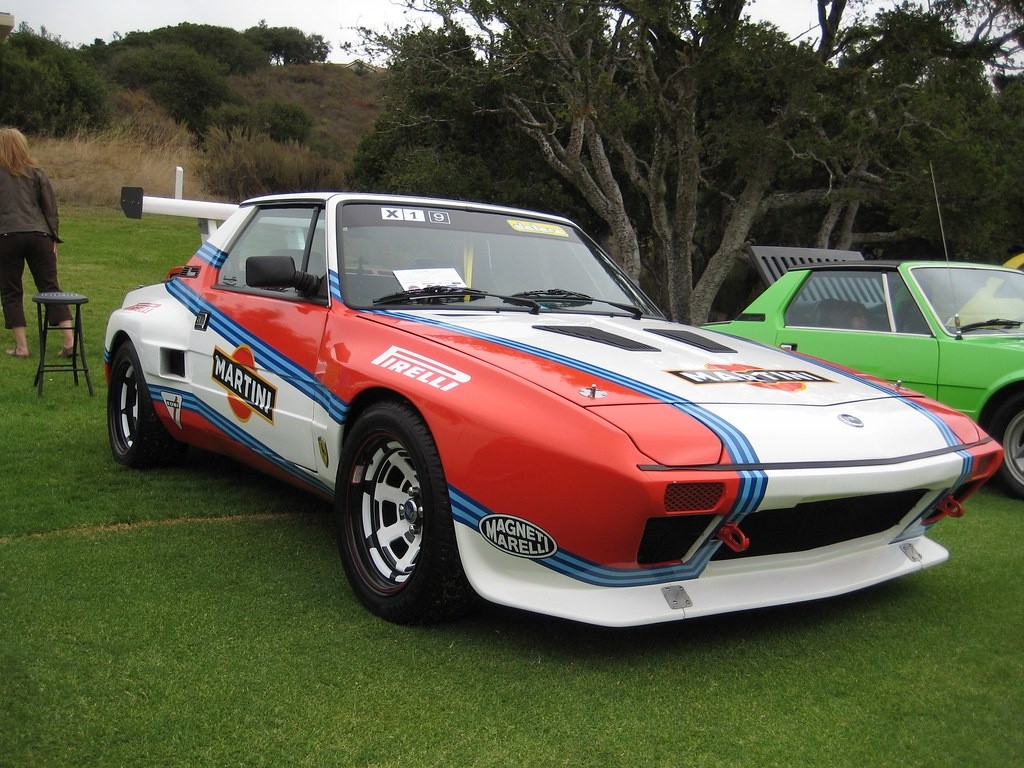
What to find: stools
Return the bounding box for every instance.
[27,294,90,398]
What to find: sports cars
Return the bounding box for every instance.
[101,166,1004,632]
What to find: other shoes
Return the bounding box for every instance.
[7,348,29,357]
[57,346,79,357]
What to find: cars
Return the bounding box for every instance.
[697,260,1024,502]
[942,252,1024,333]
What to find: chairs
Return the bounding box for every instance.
[268,249,324,299]
[814,300,871,332]
[897,300,931,332]
[415,259,467,294]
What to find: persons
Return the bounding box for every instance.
[0,128,74,359]
